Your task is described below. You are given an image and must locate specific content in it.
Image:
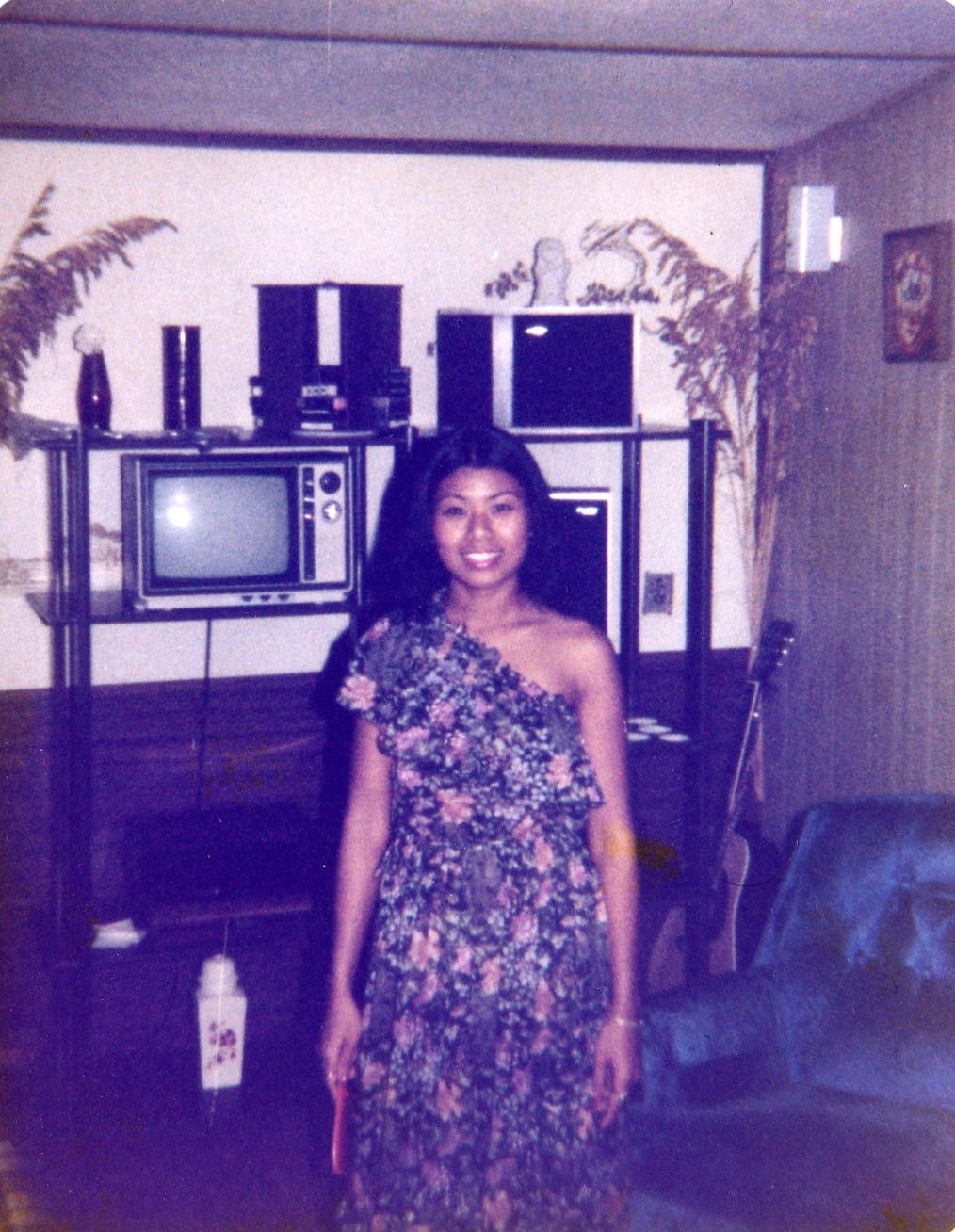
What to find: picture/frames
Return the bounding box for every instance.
[871,212,955,366]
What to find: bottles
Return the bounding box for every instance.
[75,350,111,434]
[186,954,246,1134]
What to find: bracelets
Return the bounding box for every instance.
[611,1016,641,1030]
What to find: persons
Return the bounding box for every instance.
[313,421,642,1232]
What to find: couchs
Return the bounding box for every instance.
[632,794,955,1232]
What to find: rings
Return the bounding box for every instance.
[321,1070,337,1080]
[613,1091,628,1103]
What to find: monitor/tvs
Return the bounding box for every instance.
[120,451,354,612]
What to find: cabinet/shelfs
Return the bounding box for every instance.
[27,414,733,1012]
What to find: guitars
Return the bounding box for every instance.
[644,619,796,995]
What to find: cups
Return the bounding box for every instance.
[160,324,202,433]
